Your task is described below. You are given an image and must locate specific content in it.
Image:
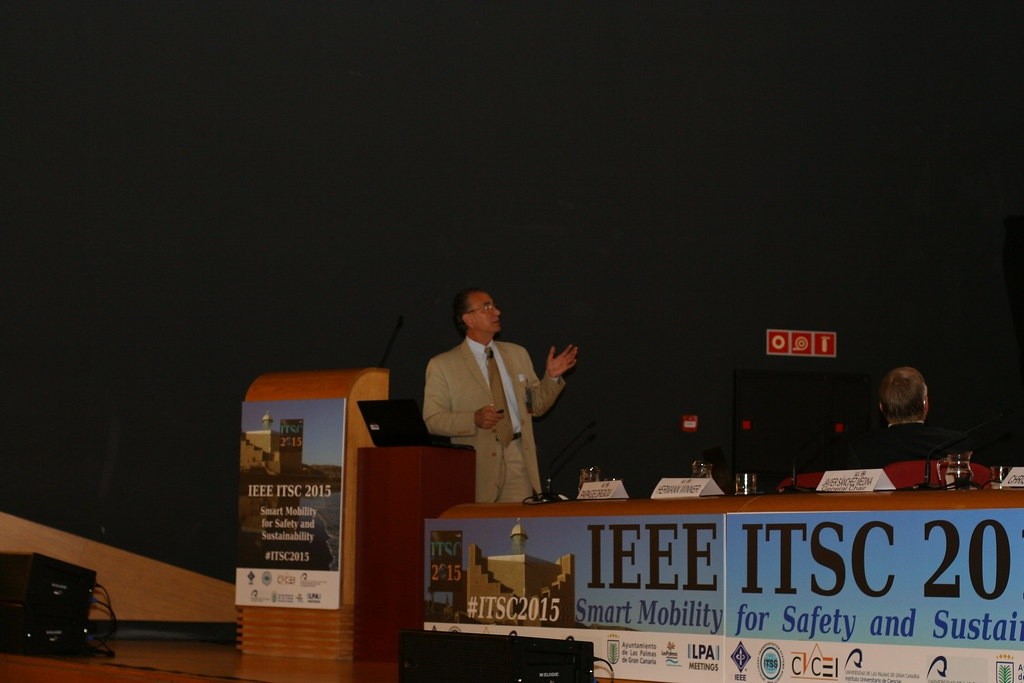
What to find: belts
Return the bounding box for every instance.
[496,432,523,442]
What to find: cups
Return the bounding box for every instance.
[990,466,1014,489]
[734,472,757,495]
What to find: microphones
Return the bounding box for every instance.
[906,410,1010,489]
[779,414,843,494]
[539,432,599,503]
[379,314,404,369]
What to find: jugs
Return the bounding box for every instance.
[937,450,974,490]
[687,460,713,482]
[577,466,602,490]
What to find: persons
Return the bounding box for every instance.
[423,288,578,504]
[853,366,972,469]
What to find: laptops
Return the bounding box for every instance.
[357,398,474,451]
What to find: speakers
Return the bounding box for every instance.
[397,628,594,682]
[0,552,97,655]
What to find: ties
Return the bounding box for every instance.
[484,346,514,449]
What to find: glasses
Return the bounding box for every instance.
[465,301,498,314]
